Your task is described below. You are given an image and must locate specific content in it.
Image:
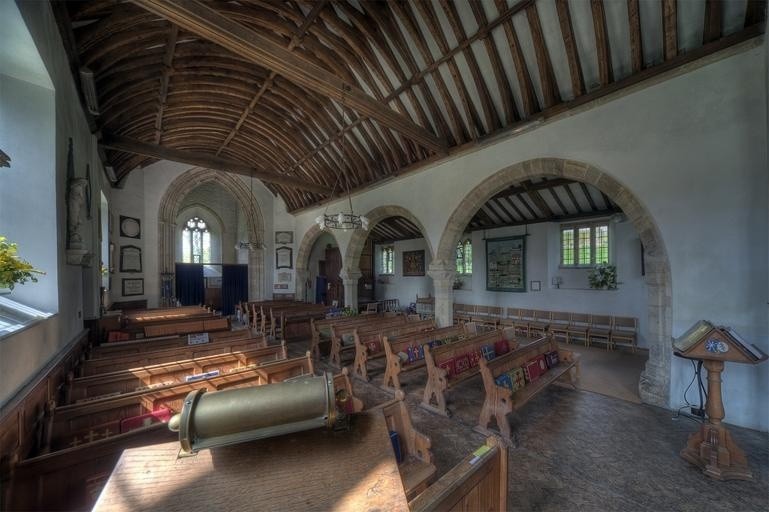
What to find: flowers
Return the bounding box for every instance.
[0,236,46,292]
[584,260,618,290]
[102,267,110,277]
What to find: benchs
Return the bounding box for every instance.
[7,299,514,511]
[478,332,580,449]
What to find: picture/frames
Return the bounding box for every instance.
[530,280,541,291]
[119,214,140,239]
[276,246,293,269]
[121,278,145,296]
[482,234,529,292]
[85,163,92,217]
[401,249,426,276]
[274,230,294,244]
[119,244,142,273]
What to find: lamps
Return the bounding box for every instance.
[231,169,269,250]
[315,85,370,232]
[105,162,118,185]
[78,65,100,118]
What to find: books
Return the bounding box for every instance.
[727,325,763,360]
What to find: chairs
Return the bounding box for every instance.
[416,294,436,318]
[501,307,639,355]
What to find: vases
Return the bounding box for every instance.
[601,284,608,291]
